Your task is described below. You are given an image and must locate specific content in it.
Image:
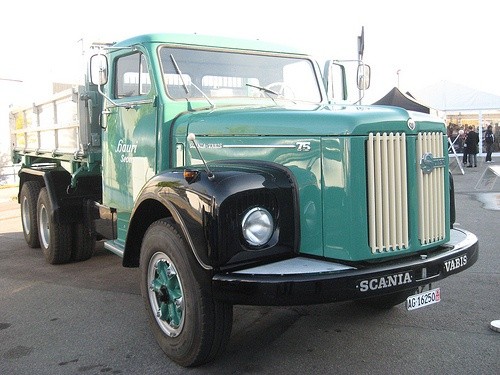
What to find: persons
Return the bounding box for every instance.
[485,125,496,163]
[463,128,471,164]
[464,126,479,167]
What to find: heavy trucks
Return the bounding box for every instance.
[7,27,478,368]
